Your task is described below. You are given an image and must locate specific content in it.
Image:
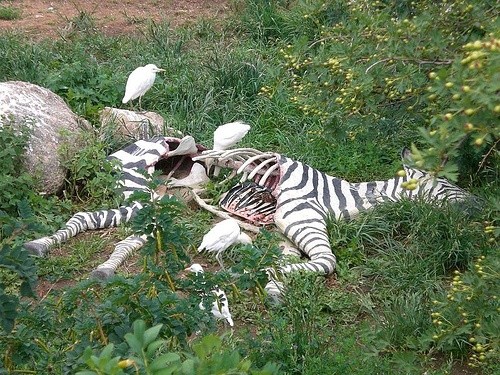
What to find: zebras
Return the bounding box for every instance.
[21,134,484,310]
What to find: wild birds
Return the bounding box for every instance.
[197,218,253,273]
[213,120,251,152]
[184,263,235,327]
[122,64,167,114]
